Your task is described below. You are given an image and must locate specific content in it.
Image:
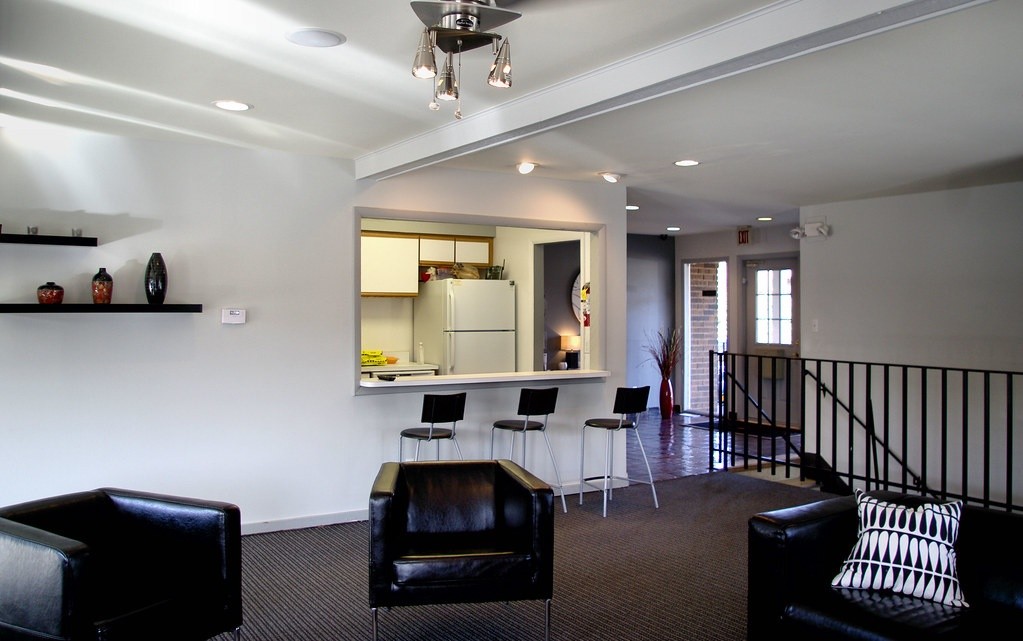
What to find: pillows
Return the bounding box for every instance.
[833,489,968,610]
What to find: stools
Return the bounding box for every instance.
[490,387,568,514]
[579,386,659,517]
[398,392,467,463]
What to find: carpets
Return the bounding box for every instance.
[680,419,800,441]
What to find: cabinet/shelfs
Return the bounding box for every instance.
[0,233,202,313]
[419,237,455,266]
[455,237,491,266]
[361,236,418,296]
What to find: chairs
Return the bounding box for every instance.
[1,487,241,640]
[368,460,553,641]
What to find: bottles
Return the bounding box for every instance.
[37,282,64,303]
[91,268,113,304]
[145,253,168,304]
[416,341,425,365]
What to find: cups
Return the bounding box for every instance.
[72,228,82,237]
[27,225,38,235]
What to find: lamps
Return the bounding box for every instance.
[603,173,621,184]
[408,1,521,122]
[559,336,581,370]
[516,163,536,173]
[790,221,832,240]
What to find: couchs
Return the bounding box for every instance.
[749,492,1023,641]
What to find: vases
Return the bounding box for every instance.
[658,379,675,420]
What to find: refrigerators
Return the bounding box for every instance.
[413,278,516,375]
